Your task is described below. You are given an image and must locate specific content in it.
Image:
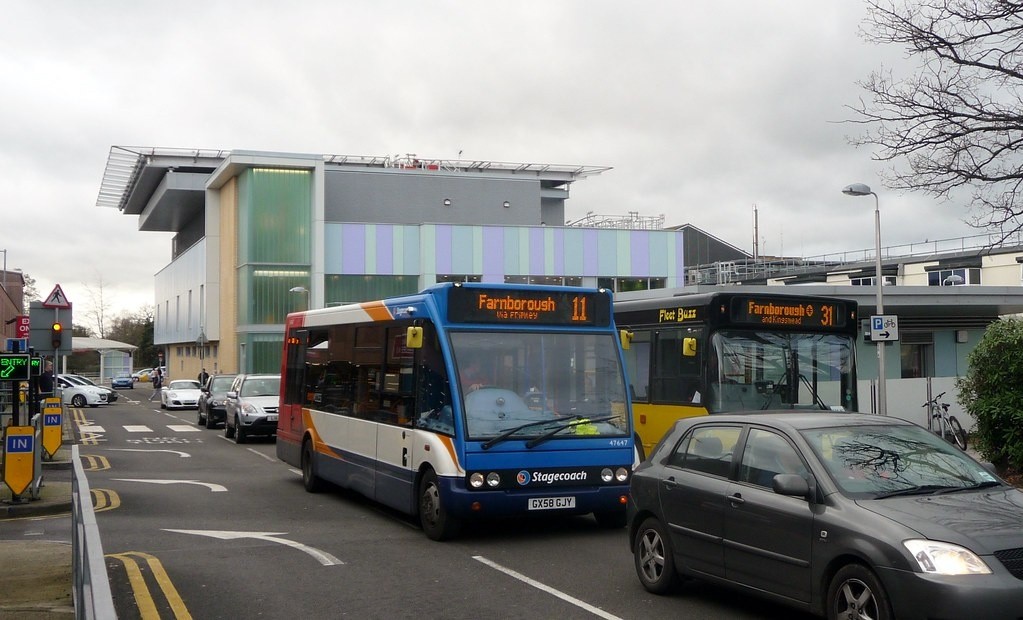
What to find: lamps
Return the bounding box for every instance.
[444,199,451,205]
[503,201,510,208]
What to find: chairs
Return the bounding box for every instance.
[679,435,725,475]
[831,436,852,477]
[303,384,416,427]
[750,436,779,485]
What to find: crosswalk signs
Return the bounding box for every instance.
[44,284,71,308]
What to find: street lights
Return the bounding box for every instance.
[290,287,310,311]
[842,183,887,417]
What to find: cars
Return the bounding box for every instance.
[148,366,167,382]
[132,368,153,382]
[197,374,238,429]
[225,374,281,445]
[110,373,135,389]
[53,374,108,408]
[71,375,118,405]
[628,410,1023,620]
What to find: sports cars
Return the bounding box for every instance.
[161,380,203,410]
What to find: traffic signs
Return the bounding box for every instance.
[870,314,899,342]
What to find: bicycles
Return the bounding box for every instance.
[922,392,968,451]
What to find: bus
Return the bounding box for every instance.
[585,292,859,461]
[277,282,633,544]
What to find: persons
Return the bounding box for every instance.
[38,360,53,399]
[199,367,209,386]
[458,352,487,392]
[149,368,162,402]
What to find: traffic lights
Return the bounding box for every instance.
[52,323,62,350]
